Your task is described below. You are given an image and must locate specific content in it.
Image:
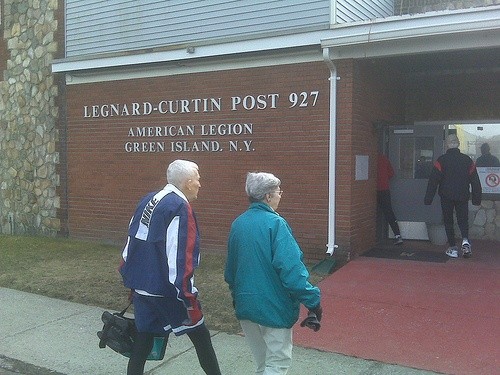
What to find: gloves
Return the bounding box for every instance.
[300,305,322,331]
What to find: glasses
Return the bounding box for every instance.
[274,191,283,195]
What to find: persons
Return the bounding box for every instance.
[424,134,482,258]
[475,143,500,167]
[225,171,323,375]
[377,151,403,245]
[119,159,221,375]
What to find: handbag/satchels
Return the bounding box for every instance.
[101,302,168,361]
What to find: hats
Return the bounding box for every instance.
[447,134,459,147]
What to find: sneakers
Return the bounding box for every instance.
[462,241,472,258]
[392,237,404,245]
[445,248,459,258]
